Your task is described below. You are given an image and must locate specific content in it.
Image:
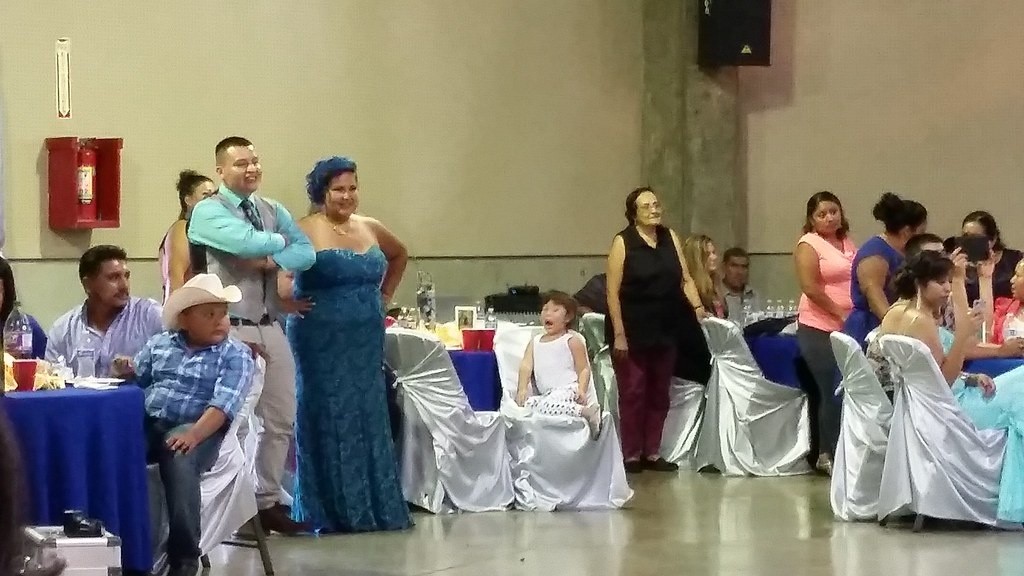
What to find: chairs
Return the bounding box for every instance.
[694,316,815,476]
[383,328,517,513]
[495,325,636,511]
[583,314,707,467]
[829,329,915,523]
[147,353,275,576]
[876,334,1004,532]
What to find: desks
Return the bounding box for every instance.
[960,357,1024,389]
[446,343,505,411]
[742,315,802,389]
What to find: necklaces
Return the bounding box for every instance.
[332,220,351,236]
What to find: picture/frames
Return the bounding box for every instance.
[454,305,477,332]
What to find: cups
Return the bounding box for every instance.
[479,330,496,352]
[462,329,479,350]
[12,361,37,392]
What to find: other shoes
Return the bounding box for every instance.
[624,455,678,474]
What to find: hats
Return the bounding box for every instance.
[161,271,245,327]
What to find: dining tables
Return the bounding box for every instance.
[6,375,153,576]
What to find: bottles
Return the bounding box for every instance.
[3,301,33,359]
[485,307,498,330]
[397,306,419,330]
[764,299,797,319]
[740,298,753,332]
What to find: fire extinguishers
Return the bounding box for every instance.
[76,137,98,220]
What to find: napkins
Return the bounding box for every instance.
[72,376,118,390]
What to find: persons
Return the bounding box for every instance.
[514,293,603,442]
[159,136,416,535]
[604,186,760,476]
[793,191,1023,532]
[0,245,253,576]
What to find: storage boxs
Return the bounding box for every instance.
[19,524,122,570]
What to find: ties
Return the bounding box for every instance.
[241,198,263,230]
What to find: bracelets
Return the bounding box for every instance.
[961,373,969,380]
[694,305,703,310]
[968,374,978,387]
